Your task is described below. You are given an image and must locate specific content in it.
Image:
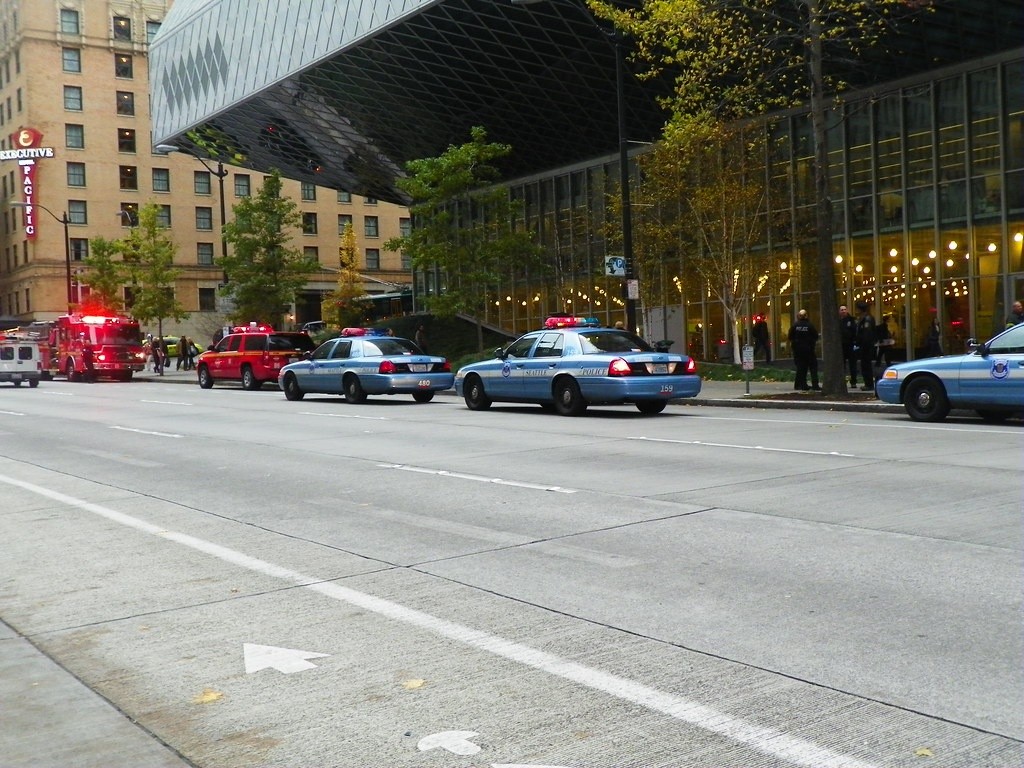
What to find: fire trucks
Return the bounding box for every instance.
[0,314,146,383]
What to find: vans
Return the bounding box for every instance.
[0,338,41,387]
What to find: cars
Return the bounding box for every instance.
[454,318,701,419]
[142,336,204,358]
[294,321,327,334]
[875,324,1024,423]
[278,328,452,404]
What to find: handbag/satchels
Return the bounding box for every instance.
[191,347,199,356]
[163,357,170,367]
[188,345,197,355]
[157,348,165,357]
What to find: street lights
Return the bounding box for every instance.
[8,202,72,314]
[154,145,230,327]
[116,211,137,307]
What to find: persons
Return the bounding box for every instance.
[386,328,394,337]
[752,313,771,365]
[1005,301,1024,330]
[143,334,198,375]
[787,306,942,391]
[415,324,427,352]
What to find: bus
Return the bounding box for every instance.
[321,289,446,332]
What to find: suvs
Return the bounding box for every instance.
[197,326,317,391]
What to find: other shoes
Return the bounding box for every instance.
[794,383,812,390]
[860,385,874,391]
[154,367,156,373]
[158,369,160,373]
[851,384,857,388]
[811,385,821,390]
